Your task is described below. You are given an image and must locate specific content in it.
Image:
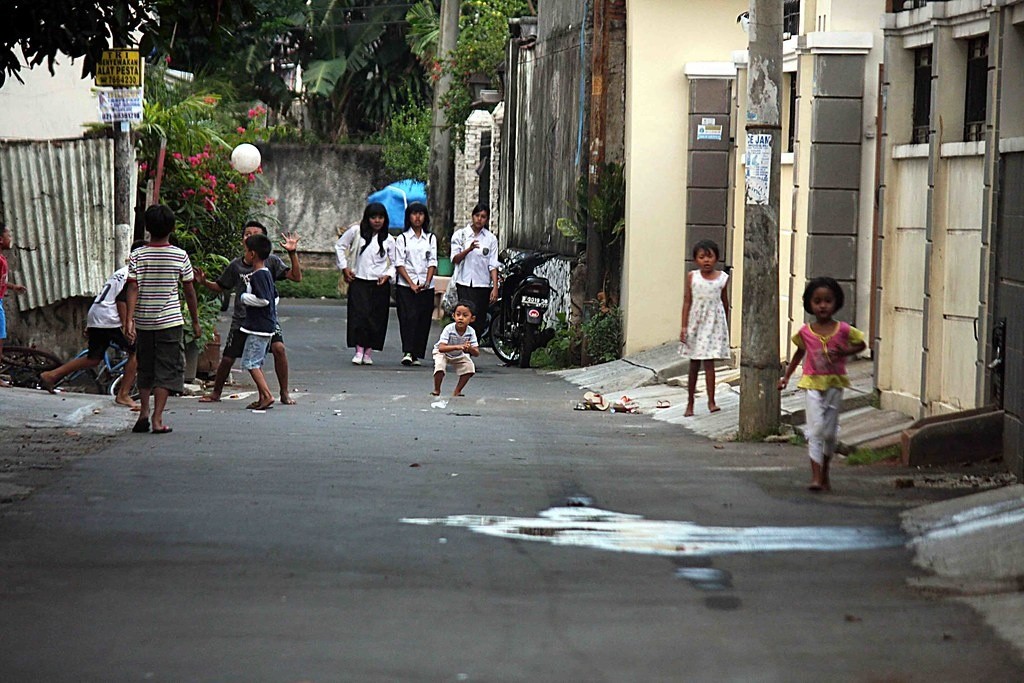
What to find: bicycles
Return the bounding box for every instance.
[0,345,64,390]
[54,339,143,401]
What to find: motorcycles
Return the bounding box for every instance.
[488,232,559,370]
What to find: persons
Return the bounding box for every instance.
[0,221,27,387]
[450,204,498,344]
[193,221,302,404]
[430,301,480,396]
[40,240,150,408]
[125,205,201,432]
[778,277,866,490]
[335,202,395,365]
[395,203,437,365]
[239,235,277,409]
[681,239,731,417]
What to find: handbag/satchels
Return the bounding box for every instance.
[338,268,352,296]
[439,279,458,315]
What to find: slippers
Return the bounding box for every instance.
[573,392,639,414]
[153,425,173,433]
[656,400,670,408]
[33,373,55,393]
[132,419,150,433]
[281,396,296,405]
[198,395,222,401]
[248,401,274,409]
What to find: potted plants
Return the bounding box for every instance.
[182,303,205,382]
[198,303,220,374]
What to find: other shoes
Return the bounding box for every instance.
[401,352,413,366]
[364,355,373,364]
[411,357,421,365]
[352,352,363,364]
